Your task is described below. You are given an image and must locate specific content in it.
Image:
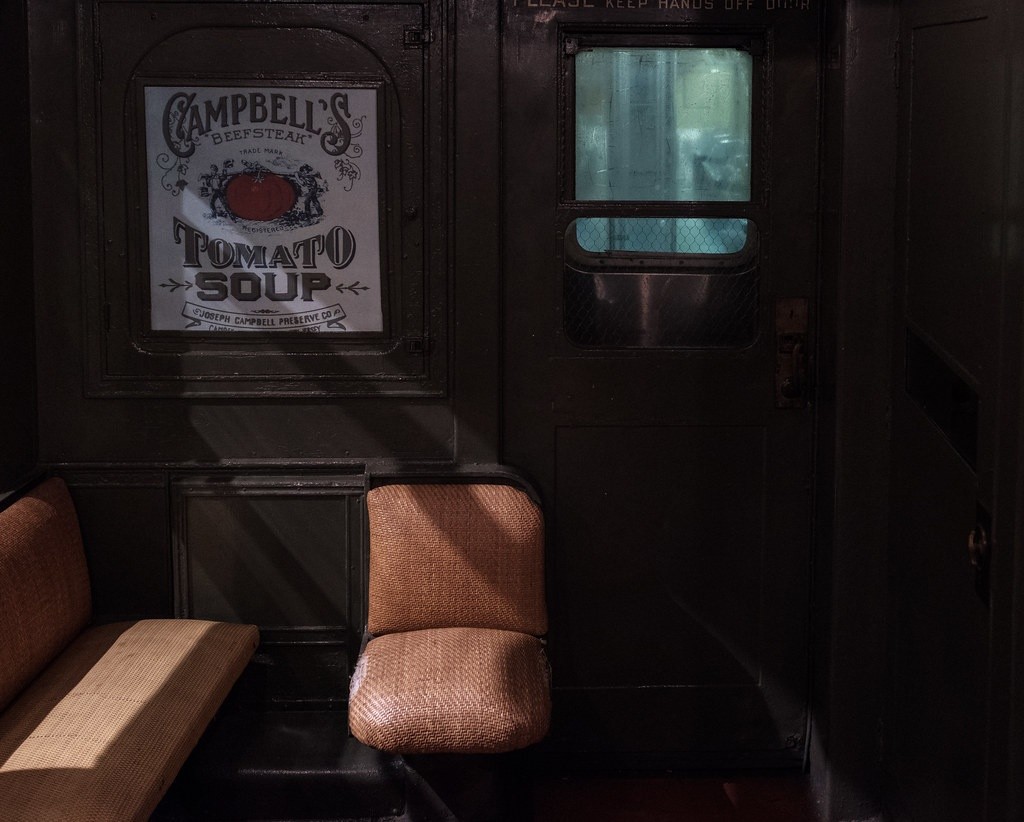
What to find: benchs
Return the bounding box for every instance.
[0,466,263,822]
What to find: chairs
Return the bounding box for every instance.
[343,463,552,822]
